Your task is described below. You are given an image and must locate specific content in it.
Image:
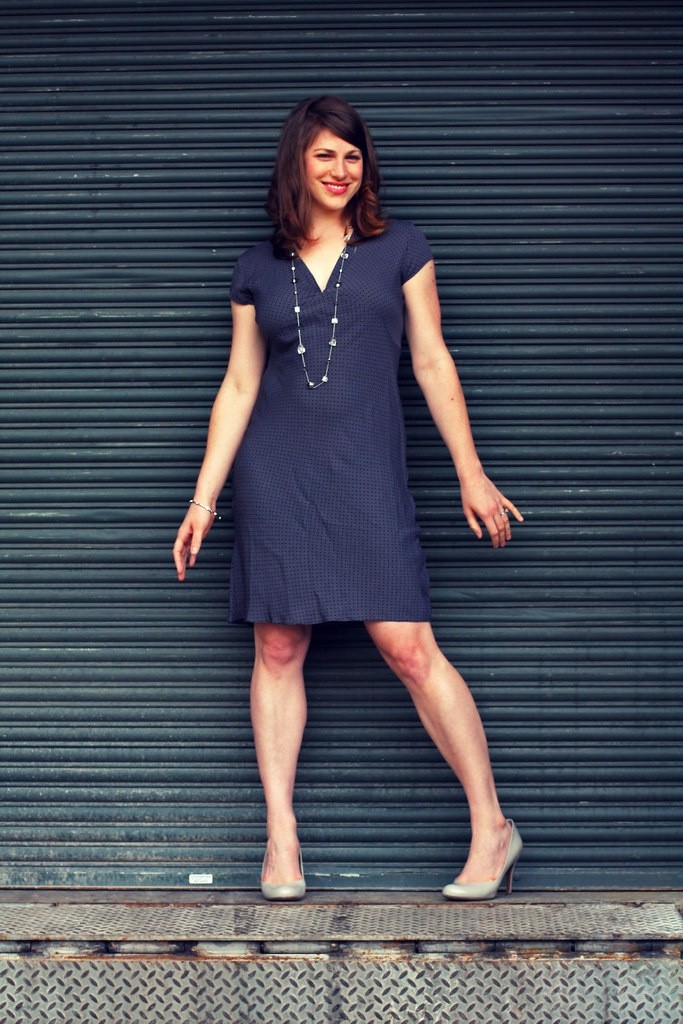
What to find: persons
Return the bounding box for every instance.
[171,96,523,898]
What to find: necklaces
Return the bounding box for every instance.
[292,230,348,391]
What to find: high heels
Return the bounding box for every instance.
[261,844,306,900]
[441,819,522,901]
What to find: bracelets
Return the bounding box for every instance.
[189,500,221,520]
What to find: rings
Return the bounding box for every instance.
[500,509,509,516]
